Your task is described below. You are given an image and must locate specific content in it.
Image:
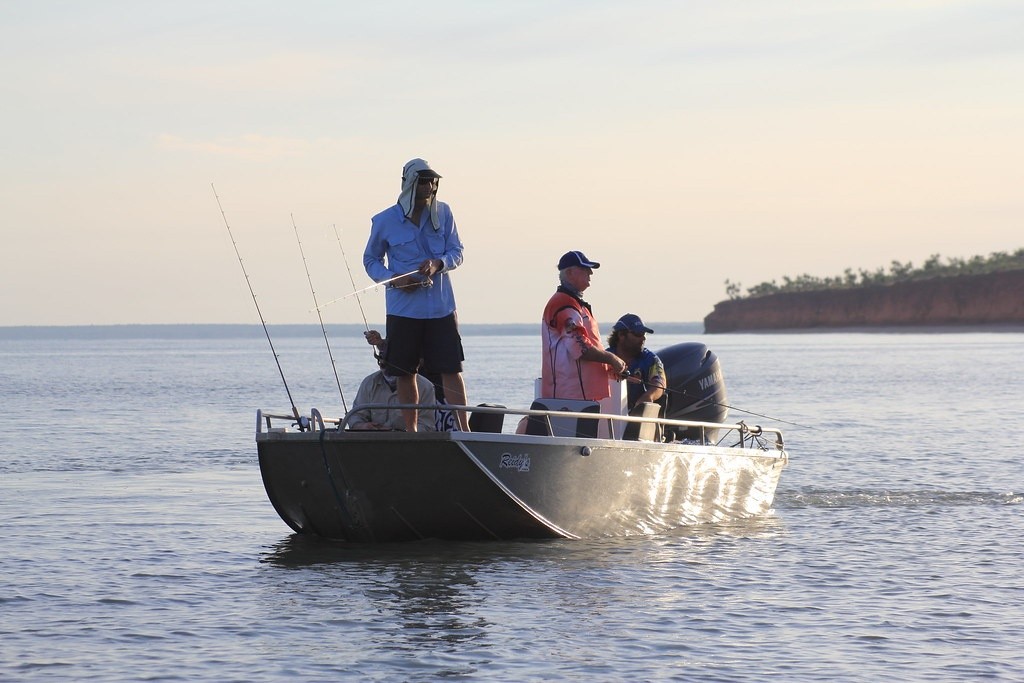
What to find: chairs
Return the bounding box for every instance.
[525,398,601,437]
[623,401,661,442]
[468,404,507,433]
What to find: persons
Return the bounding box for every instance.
[348,330,458,431]
[603,313,676,443]
[541,251,628,399]
[364,159,468,432]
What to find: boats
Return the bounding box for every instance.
[256,404,790,541]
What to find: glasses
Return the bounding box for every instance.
[377,360,384,369]
[622,330,645,336]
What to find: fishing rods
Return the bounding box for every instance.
[308,268,421,314]
[333,223,378,359]
[211,183,305,433]
[290,212,348,415]
[620,371,827,432]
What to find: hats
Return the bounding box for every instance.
[397,157,443,232]
[613,313,654,333]
[557,250,601,271]
